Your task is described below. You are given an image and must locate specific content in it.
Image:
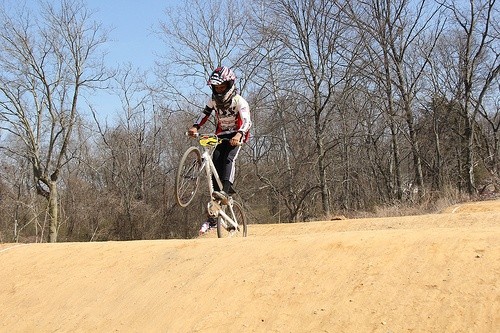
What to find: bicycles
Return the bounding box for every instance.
[176,132,247,238]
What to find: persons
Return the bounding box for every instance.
[185,65,252,231]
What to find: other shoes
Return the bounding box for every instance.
[212,190,230,205]
[207,215,217,226]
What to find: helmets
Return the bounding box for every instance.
[206,66,237,106]
